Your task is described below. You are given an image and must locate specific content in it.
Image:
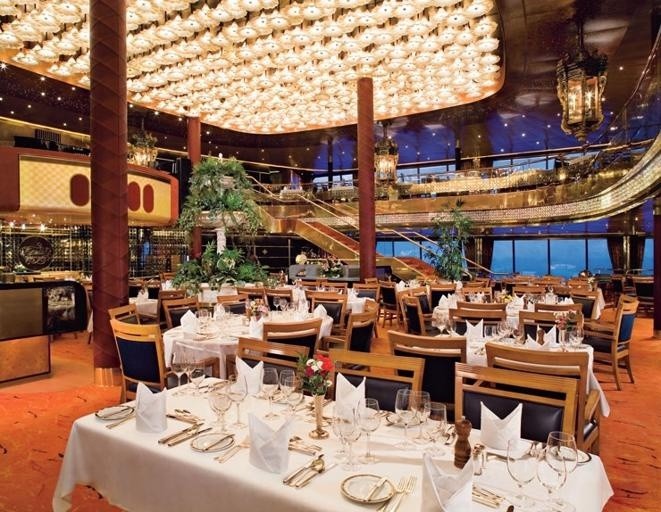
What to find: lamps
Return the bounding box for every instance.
[368,114,403,187]
[548,0,616,148]
[0,2,513,143]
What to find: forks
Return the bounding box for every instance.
[375,475,418,511]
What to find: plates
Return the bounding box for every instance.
[322,404,375,421]
[95,404,133,421]
[487,439,533,460]
[342,472,396,504]
[190,432,236,451]
[549,443,592,464]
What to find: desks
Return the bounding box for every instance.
[51,376,616,511]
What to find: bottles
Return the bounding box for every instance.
[451,418,487,475]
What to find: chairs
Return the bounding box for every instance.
[0,258,655,456]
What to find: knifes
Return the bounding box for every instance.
[363,475,389,503]
[282,453,325,483]
[168,426,214,447]
[297,461,338,489]
[159,421,202,444]
[203,433,234,451]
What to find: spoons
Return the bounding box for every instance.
[289,457,324,488]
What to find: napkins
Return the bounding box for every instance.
[247,413,295,473]
[423,453,476,511]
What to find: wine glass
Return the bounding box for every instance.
[332,387,448,469]
[167,350,303,430]
[468,286,564,305]
[434,308,586,351]
[505,430,580,512]
[195,298,312,338]
